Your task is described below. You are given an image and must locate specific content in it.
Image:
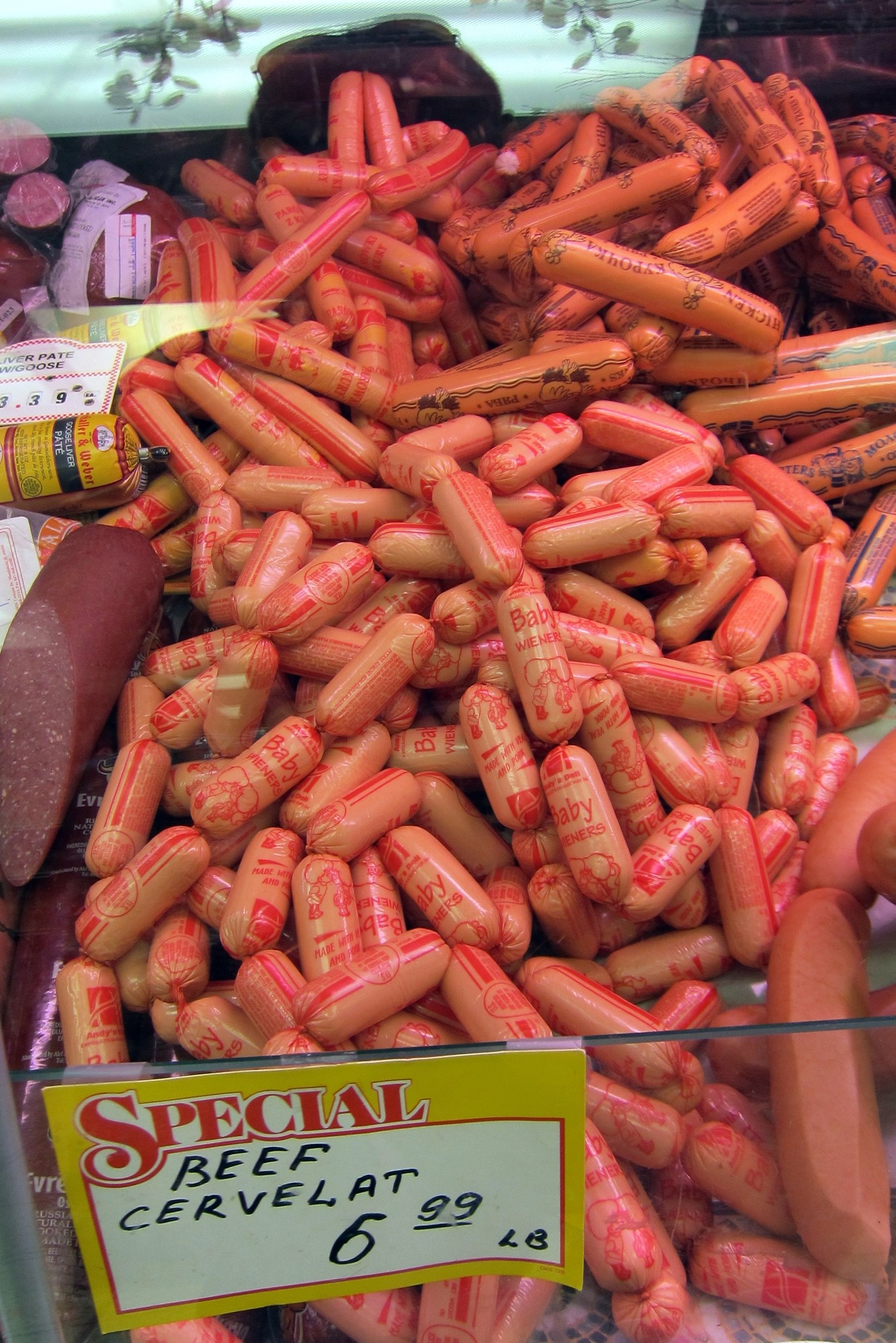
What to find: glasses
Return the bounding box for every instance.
[253,14,456,95]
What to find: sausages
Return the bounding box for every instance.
[0,54,896,1343]
